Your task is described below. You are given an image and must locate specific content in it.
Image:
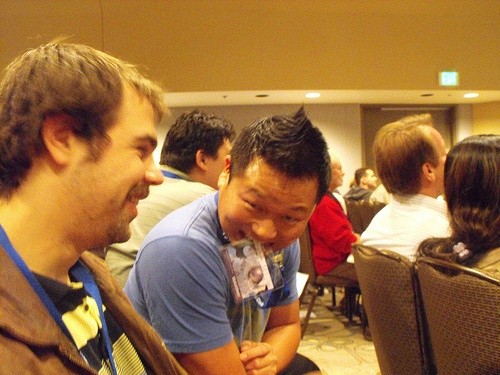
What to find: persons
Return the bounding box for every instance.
[309,153,362,278]
[343,168,391,204]
[121,107,332,375]
[356,112,457,262]
[104,111,237,290]
[1,40,189,375]
[416,134,500,280]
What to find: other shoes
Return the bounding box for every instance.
[339,297,360,313]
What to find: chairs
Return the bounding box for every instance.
[298,219,365,340]
[352,244,500,375]
[79,251,188,375]
